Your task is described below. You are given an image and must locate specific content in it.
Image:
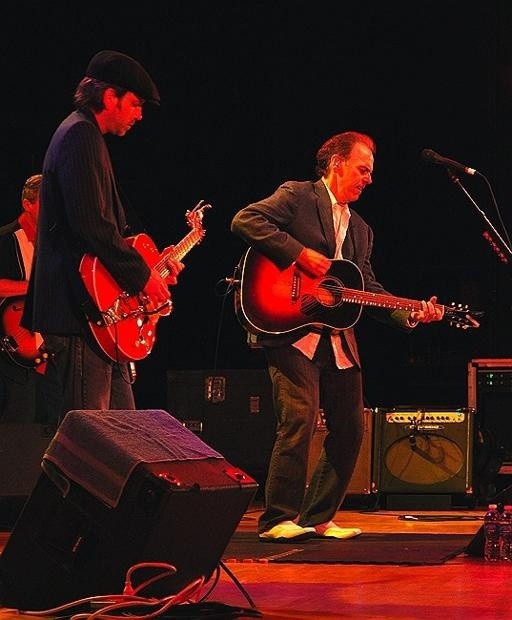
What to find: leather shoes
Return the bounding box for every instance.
[258,521,316,542]
[314,521,362,540]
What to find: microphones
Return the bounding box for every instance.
[408,426,417,453]
[421,148,480,176]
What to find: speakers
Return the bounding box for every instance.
[303,408,373,495]
[1,409,259,615]
[465,359,512,470]
[371,406,472,493]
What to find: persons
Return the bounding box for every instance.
[24,51,185,423]
[0,172,66,444]
[229,128,443,544]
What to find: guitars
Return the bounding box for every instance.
[234,245,480,339]
[0,295,52,368]
[79,198,212,365]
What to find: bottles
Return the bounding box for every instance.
[483,503,511,563]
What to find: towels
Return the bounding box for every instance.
[43,407,226,507]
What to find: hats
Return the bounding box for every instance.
[86,50,160,106]
